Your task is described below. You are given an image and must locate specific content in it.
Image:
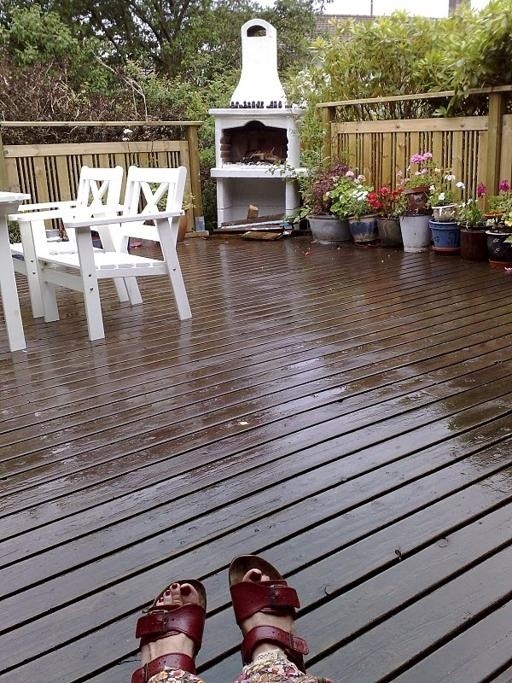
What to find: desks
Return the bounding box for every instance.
[0,192,31,353]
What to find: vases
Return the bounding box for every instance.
[483,211,512,262]
[377,214,404,247]
[347,215,378,244]
[405,186,434,215]
[430,203,458,222]
[460,221,493,264]
[429,217,462,249]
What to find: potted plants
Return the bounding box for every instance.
[309,160,353,242]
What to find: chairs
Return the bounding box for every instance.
[8,166,130,318]
[18,166,193,342]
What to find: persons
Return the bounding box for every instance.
[131,554,334,683]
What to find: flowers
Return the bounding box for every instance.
[478,180,512,212]
[366,187,404,219]
[397,152,454,187]
[322,171,374,221]
[429,174,456,206]
[438,181,484,226]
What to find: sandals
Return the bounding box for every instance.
[131,580,207,683]
[229,554,309,674]
